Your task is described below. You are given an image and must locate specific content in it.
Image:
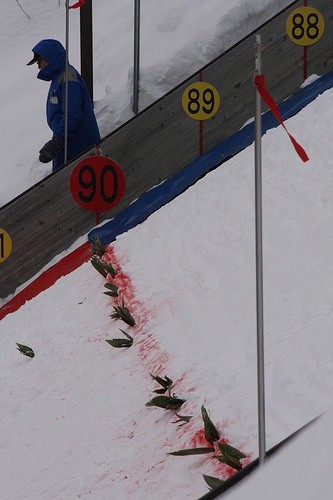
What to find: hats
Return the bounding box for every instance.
[26,51,41,66]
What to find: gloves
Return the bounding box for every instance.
[38,136,66,163]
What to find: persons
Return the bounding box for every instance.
[26,39,100,173]
